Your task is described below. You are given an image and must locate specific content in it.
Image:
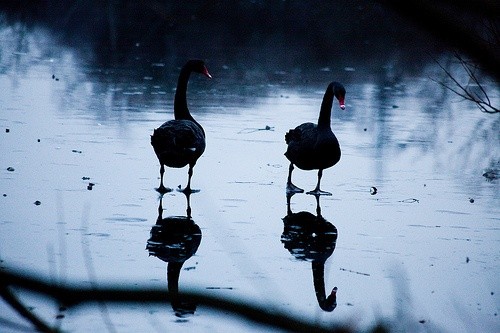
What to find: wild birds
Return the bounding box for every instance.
[284,80,346,196]
[280,195,338,312]
[144,191,202,315]
[150,59,212,191]
